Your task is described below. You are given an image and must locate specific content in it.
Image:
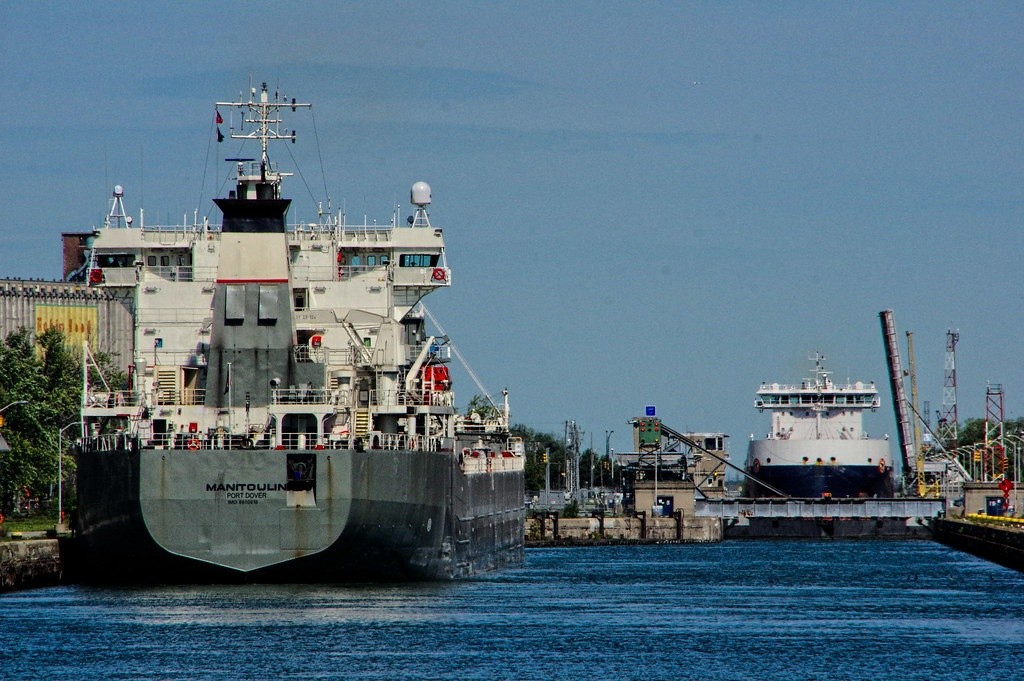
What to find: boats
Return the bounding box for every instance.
[744,349,899,538]
[81,72,527,583]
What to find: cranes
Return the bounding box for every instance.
[906,330,947,496]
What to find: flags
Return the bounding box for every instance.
[216,111,223,124]
[217,127,224,143]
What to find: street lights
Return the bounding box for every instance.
[611,448,614,493]
[59,421,82,525]
[606,430,615,490]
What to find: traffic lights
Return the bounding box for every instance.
[564,420,578,496]
[543,452,547,464]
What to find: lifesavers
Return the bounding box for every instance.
[91,270,102,283]
[433,268,444,280]
[187,439,199,450]
